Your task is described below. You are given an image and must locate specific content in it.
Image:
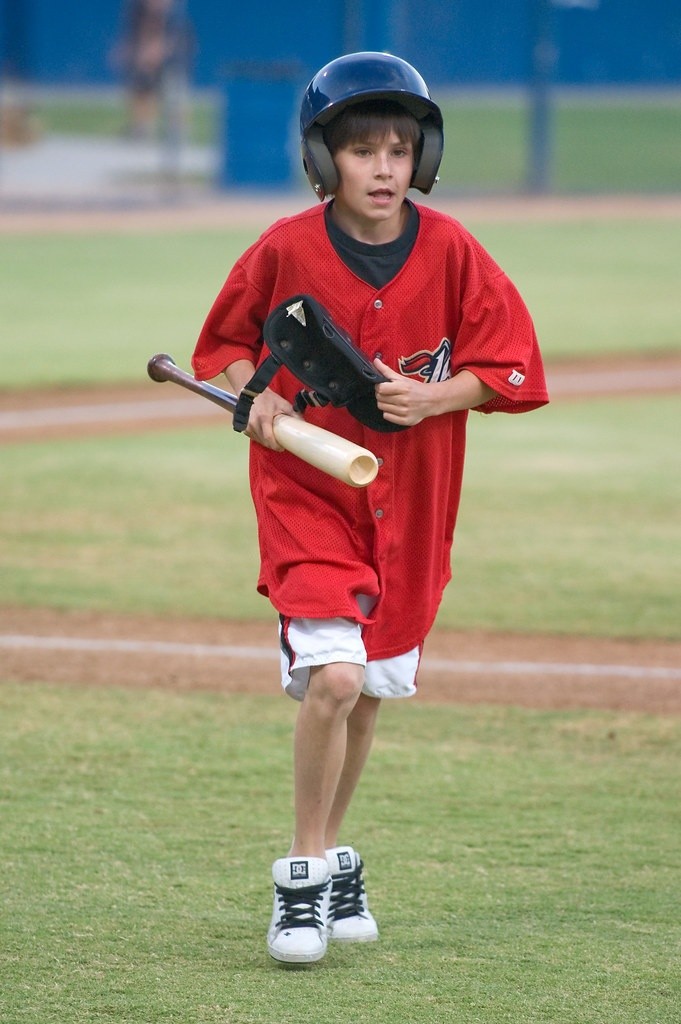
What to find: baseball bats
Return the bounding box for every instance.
[146,351,381,490]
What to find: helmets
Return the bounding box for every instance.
[300,51,445,203]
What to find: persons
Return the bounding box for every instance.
[191,50,551,963]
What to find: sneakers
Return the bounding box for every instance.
[325,846,379,943]
[266,857,333,963]
